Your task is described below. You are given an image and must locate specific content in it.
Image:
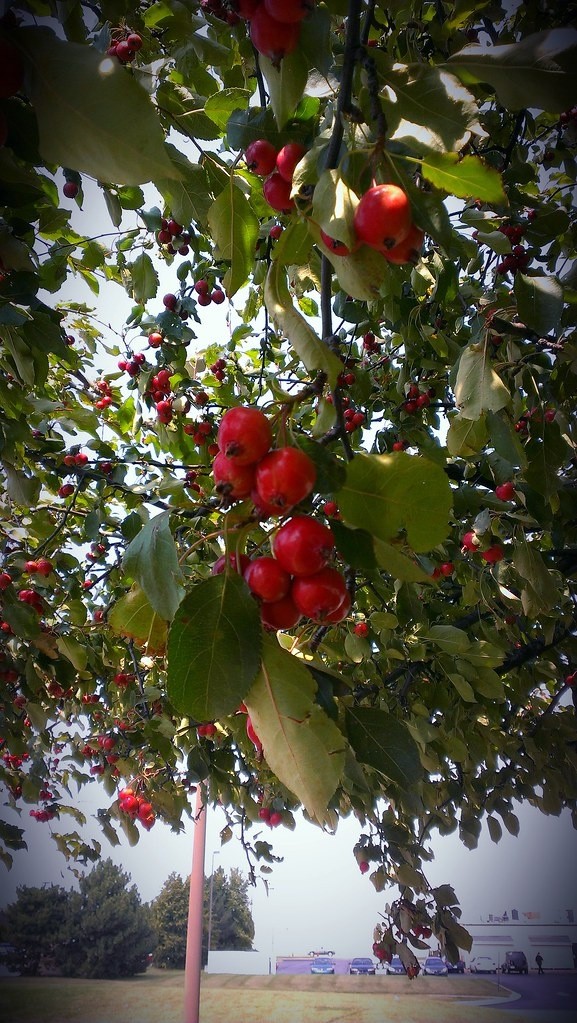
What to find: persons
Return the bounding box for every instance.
[536,951,546,976]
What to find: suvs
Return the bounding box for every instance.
[500,950,529,975]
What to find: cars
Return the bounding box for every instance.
[310,957,336,974]
[422,956,449,976]
[444,958,466,974]
[348,957,377,975]
[385,956,408,976]
[307,946,336,957]
[469,956,497,974]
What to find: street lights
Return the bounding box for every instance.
[207,849,222,951]
